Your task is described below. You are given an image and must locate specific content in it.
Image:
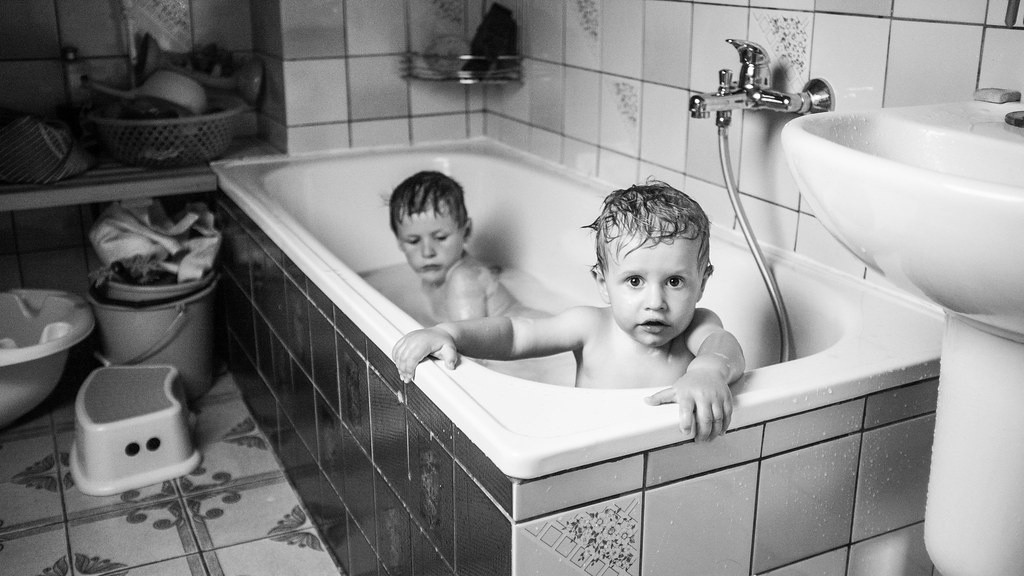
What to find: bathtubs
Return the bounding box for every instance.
[211,132,946,576]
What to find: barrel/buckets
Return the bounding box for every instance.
[107,271,216,303]
[90,276,218,404]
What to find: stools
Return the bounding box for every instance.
[70,365,200,496]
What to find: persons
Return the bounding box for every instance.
[392,176,745,444]
[389,170,557,321]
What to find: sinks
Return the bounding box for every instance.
[776,106,1024,311]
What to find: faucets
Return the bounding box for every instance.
[689,35,773,122]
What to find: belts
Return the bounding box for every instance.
[85,90,254,171]
